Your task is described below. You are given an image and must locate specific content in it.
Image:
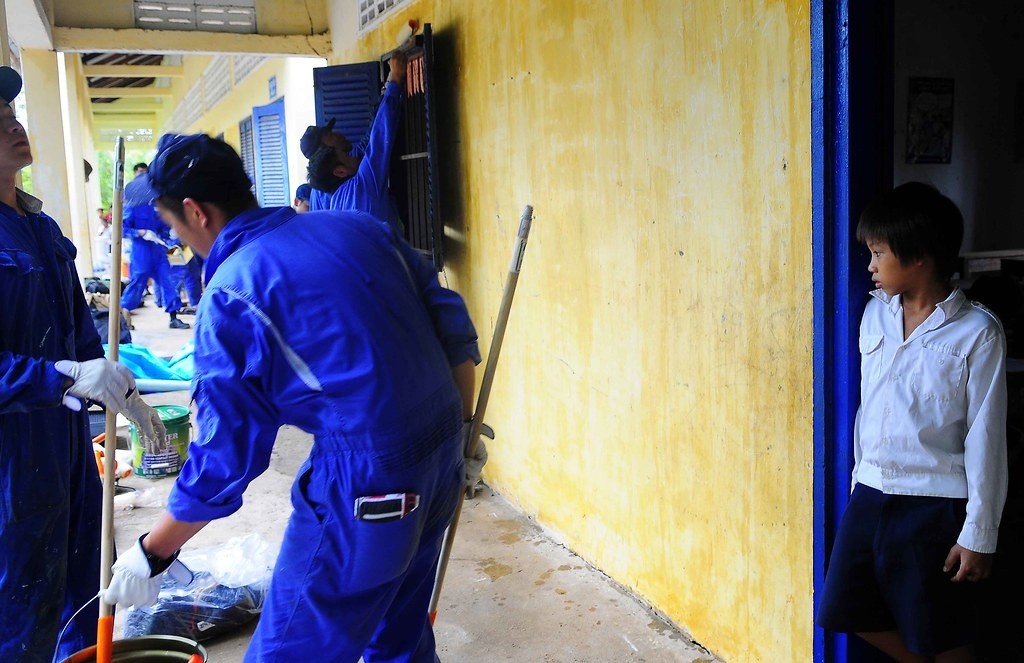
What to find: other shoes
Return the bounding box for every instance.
[176,307,193,314]
[463,478,482,499]
[170,318,190,329]
[182,303,187,307]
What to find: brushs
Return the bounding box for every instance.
[155,238,184,259]
[390,19,419,56]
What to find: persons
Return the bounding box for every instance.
[114,53,413,331]
[816,179,1008,662]
[0,64,170,662]
[102,132,488,663]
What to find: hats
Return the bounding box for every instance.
[300,117,336,158]
[0,66,22,103]
[296,183,311,202]
[121,133,209,208]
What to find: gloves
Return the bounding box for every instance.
[460,419,495,486]
[103,531,194,610]
[54,357,136,416]
[118,386,166,455]
[169,228,181,241]
[142,229,165,246]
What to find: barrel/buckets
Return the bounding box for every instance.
[127,404,194,479]
[52,590,208,663]
